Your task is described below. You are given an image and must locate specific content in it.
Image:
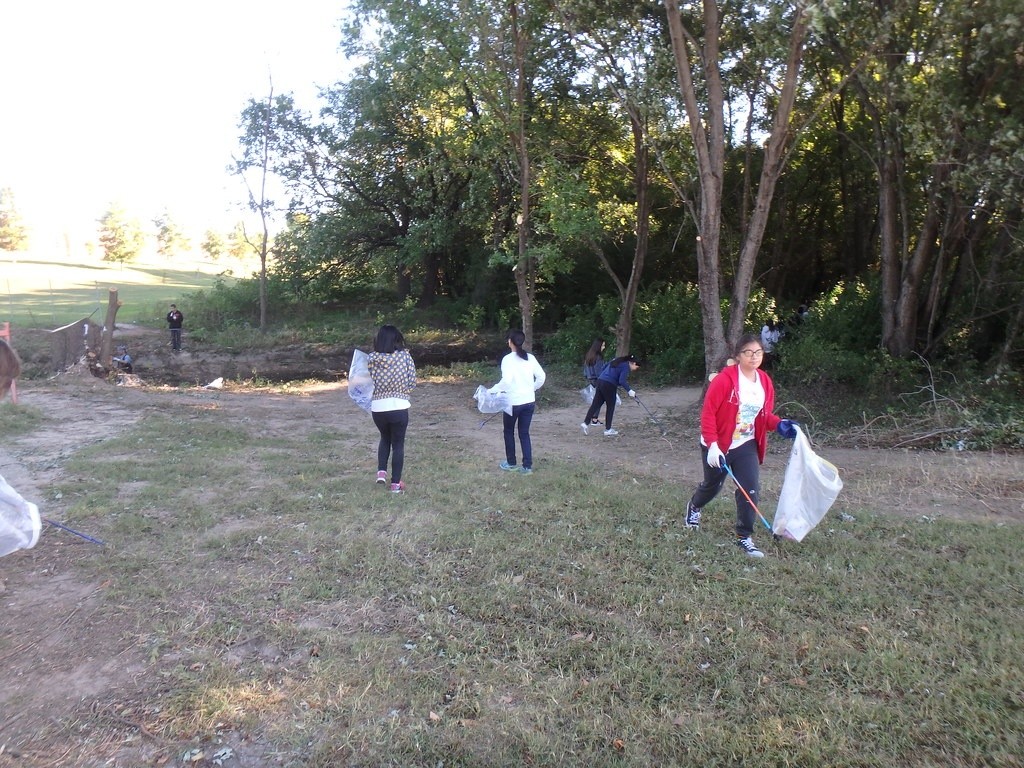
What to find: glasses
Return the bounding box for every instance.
[740,349,765,357]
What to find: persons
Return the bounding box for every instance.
[685,332,800,558]
[366,323,417,494]
[580,354,641,436]
[486,328,546,475]
[167,304,183,351]
[761,304,810,372]
[112,345,132,374]
[583,337,606,426]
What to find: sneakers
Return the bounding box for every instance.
[581,422,589,435]
[517,465,533,475]
[736,535,764,558]
[500,461,519,471]
[604,428,618,435]
[376,470,387,484]
[390,480,404,493]
[685,499,701,530]
[591,419,603,425]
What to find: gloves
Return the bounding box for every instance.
[707,442,726,473]
[777,418,800,439]
[628,390,636,397]
